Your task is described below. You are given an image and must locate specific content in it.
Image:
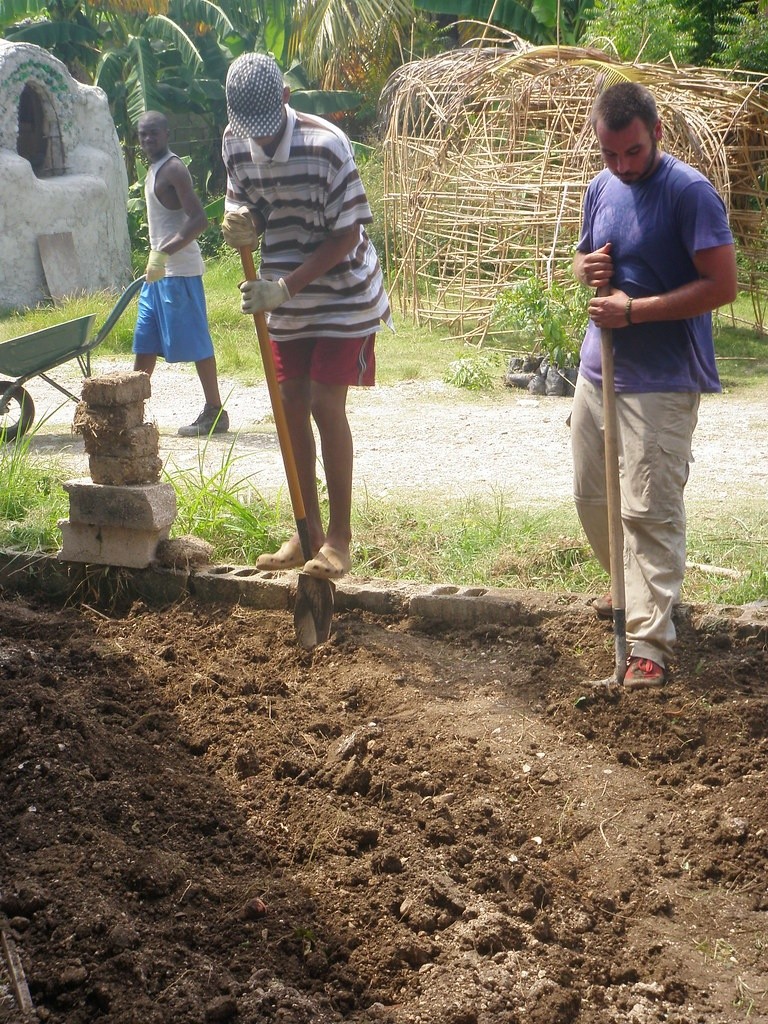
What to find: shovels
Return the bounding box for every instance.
[593,280,638,686]
[231,244,336,654]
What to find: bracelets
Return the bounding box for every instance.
[624,296,634,327]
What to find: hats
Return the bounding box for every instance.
[226,53,284,135]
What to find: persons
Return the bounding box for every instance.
[220,53,398,578]
[565,80,738,690]
[133,111,230,437]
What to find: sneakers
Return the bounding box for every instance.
[177,404,229,435]
[624,656,665,688]
[592,593,612,615]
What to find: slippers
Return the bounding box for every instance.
[303,544,353,579]
[255,542,304,571]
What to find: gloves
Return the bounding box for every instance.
[221,205,254,247]
[144,250,170,284]
[237,276,291,314]
